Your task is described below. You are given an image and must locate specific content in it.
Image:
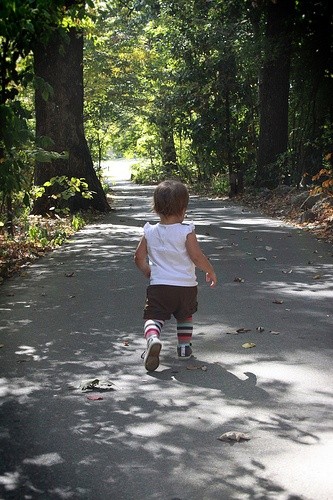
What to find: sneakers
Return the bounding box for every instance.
[177,343,192,360]
[141,335,162,372]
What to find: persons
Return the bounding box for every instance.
[134,180,217,371]
